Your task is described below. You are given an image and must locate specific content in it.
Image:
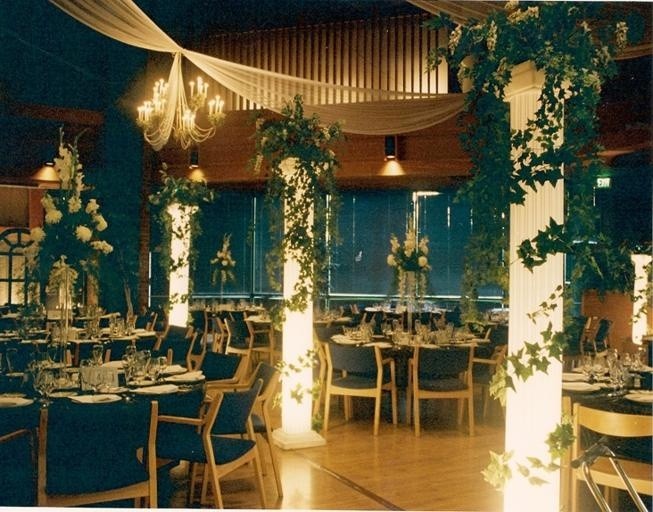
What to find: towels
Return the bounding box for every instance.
[174,370,203,381]
[128,384,179,395]
[65,394,118,404]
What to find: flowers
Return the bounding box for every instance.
[17,124,114,292]
[387,215,431,317]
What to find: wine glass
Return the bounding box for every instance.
[74,342,115,401]
[380,316,454,348]
[582,349,648,398]
[123,346,167,385]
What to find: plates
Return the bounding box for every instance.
[49,391,76,398]
[624,392,653,403]
[102,360,129,374]
[163,375,204,382]
[562,383,591,392]
[102,386,129,393]
[563,373,585,381]
[136,384,177,396]
[165,366,186,374]
[0,397,33,408]
[70,394,122,403]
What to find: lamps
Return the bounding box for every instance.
[383,135,399,160]
[629,253,652,345]
[190,146,199,169]
[41,143,55,166]
[136,53,226,151]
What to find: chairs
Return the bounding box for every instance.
[564,313,612,369]
[189,361,283,505]
[189,303,281,364]
[571,402,652,512]
[145,377,267,508]
[457,324,508,425]
[406,338,477,438]
[199,352,248,385]
[73,314,198,366]
[339,305,459,333]
[313,327,350,421]
[324,341,398,436]
[37,398,159,508]
